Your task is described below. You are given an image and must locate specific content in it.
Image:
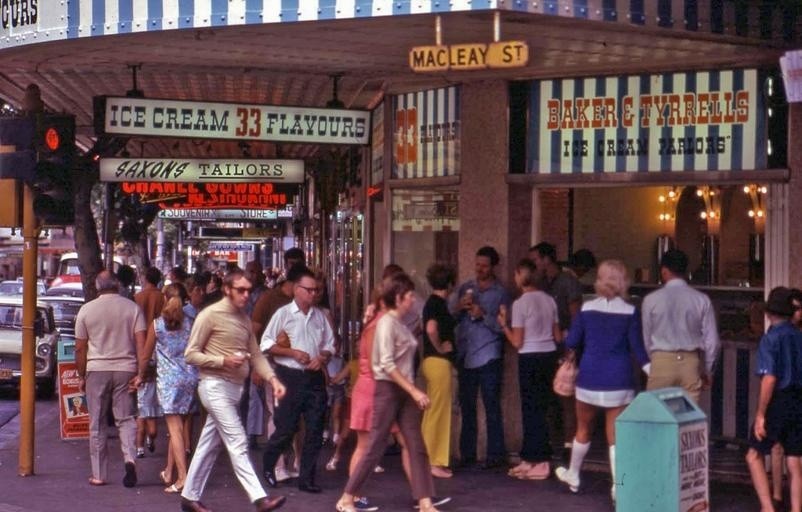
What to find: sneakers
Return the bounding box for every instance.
[335,496,378,512]
[414,495,452,509]
[430,457,506,478]
[263,464,300,487]
[321,437,339,470]
[89,436,154,487]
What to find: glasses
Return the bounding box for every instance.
[297,285,319,294]
[230,287,253,294]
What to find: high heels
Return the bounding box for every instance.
[556,466,580,493]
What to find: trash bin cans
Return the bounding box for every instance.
[57,339,91,440]
[613,386,709,511]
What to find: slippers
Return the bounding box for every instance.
[506,465,548,480]
[160,471,182,493]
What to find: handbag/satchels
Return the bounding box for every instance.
[553,359,579,397]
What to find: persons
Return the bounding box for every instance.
[641,252,723,405]
[742,285,801,512]
[768,287,801,512]
[551,258,648,508]
[71,242,599,512]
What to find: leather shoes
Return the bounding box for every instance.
[299,484,321,492]
[182,497,212,512]
[255,495,286,512]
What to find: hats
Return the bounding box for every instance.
[757,287,801,314]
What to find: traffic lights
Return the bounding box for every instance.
[1,117,36,185]
[34,113,75,224]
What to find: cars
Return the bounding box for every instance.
[1,248,128,398]
